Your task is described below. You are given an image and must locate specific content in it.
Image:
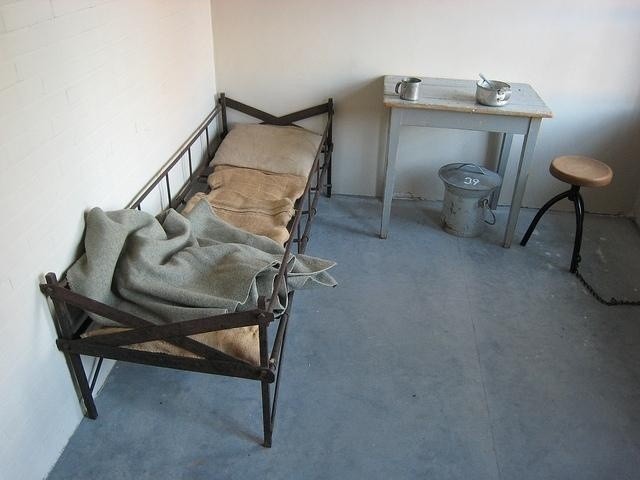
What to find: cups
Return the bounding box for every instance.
[395,78,422,100]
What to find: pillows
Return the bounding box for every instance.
[209,122,323,177]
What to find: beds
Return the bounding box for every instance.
[39,93,332,447]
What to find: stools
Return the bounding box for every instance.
[520,156,613,273]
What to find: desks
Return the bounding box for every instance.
[380,74,552,248]
